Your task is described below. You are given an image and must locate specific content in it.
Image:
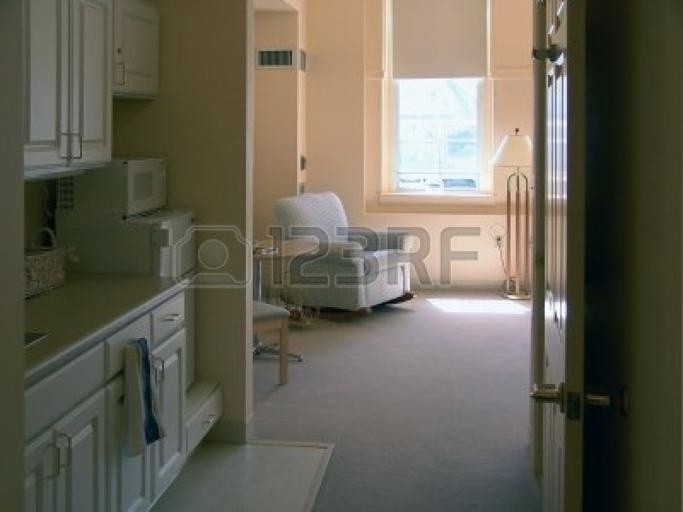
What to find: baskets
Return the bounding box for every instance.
[25,228,66,299]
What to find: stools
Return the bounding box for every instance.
[253,296,295,386]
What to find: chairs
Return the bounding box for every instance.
[267,192,419,324]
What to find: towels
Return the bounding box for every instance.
[134,334,166,445]
[121,340,149,458]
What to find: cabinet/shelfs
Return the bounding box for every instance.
[149,288,192,509]
[184,380,225,459]
[104,309,149,511]
[22,337,104,511]
[23,1,116,182]
[112,0,161,104]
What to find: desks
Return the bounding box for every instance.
[251,238,322,328]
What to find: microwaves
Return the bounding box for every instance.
[55,159,168,216]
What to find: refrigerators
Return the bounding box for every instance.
[51,213,199,395]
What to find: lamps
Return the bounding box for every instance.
[490,126,537,302]
